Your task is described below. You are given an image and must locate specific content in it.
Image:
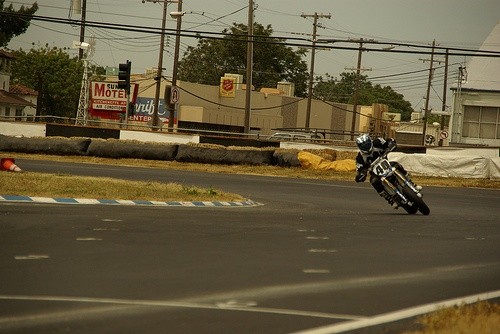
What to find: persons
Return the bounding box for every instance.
[356,133,422,210]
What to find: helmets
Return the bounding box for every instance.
[355,133,374,155]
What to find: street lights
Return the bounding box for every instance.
[169,10,253,133]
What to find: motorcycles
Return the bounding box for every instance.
[358,144,430,215]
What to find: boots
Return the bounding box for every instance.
[404,172,423,190]
[378,190,398,208]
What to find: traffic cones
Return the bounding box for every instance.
[0,157,22,172]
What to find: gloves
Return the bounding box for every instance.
[387,142,397,151]
[355,174,366,183]
